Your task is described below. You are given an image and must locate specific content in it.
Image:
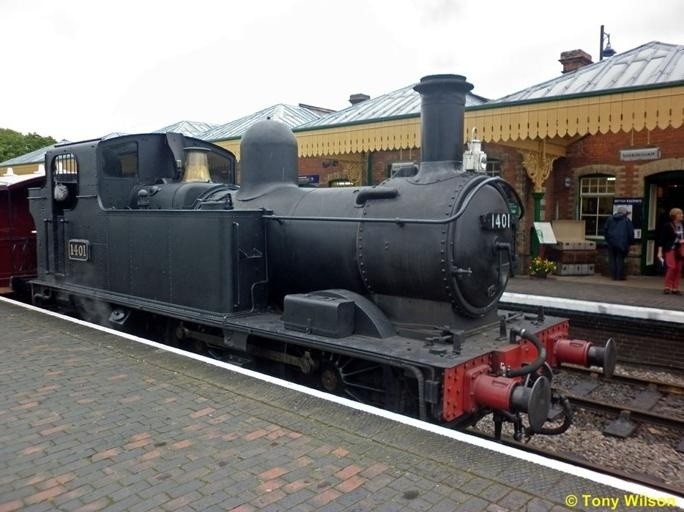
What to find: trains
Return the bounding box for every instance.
[0,74,618,442]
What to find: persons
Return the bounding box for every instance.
[602,207,635,281]
[657,208,684,295]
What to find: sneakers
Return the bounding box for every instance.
[663,288,681,295]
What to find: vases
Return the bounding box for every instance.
[536,271,546,278]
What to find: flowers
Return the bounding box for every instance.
[528,256,557,274]
